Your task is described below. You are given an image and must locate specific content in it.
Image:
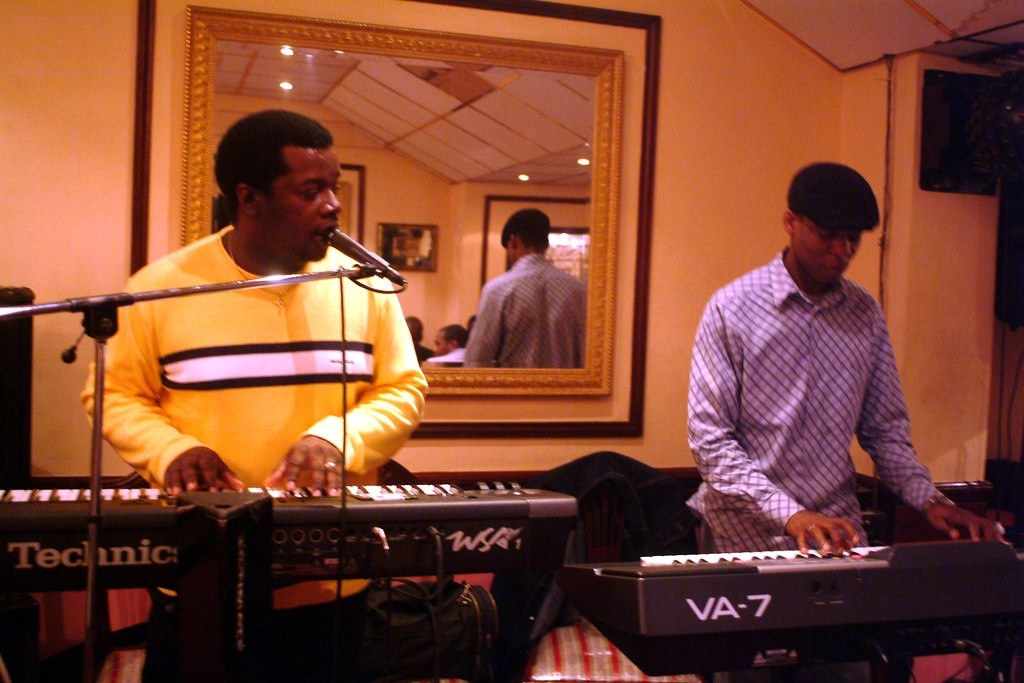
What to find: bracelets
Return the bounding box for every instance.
[923,494,956,514]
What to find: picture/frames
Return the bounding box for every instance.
[336,161,366,246]
[376,221,440,272]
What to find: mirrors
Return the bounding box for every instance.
[131,0,663,438]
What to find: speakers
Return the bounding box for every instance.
[919,68,1006,196]
[0,286,35,490]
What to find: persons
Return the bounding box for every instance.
[404,316,436,365]
[466,208,588,374]
[683,162,1010,681]
[80,109,428,683]
[435,326,468,368]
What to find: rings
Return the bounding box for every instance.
[807,522,820,533]
[323,461,338,472]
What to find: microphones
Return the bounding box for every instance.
[329,229,407,287]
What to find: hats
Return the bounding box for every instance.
[500,208,550,246]
[788,162,880,229]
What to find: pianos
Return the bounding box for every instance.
[0,477,583,602]
[559,537,1024,679]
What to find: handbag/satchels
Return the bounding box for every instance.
[364,580,502,679]
